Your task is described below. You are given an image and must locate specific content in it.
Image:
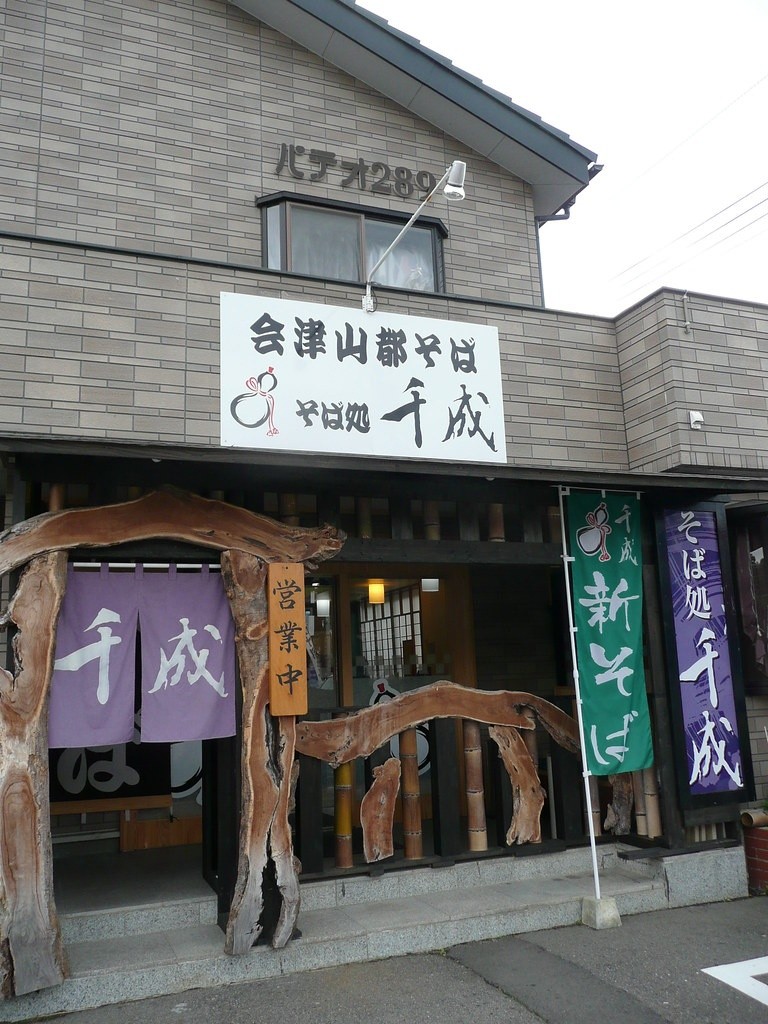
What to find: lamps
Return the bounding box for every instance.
[317,600,330,617]
[369,583,384,604]
[361,160,467,312]
[422,579,439,592]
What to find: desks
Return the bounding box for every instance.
[553,685,614,789]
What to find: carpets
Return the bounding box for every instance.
[288,811,402,858]
